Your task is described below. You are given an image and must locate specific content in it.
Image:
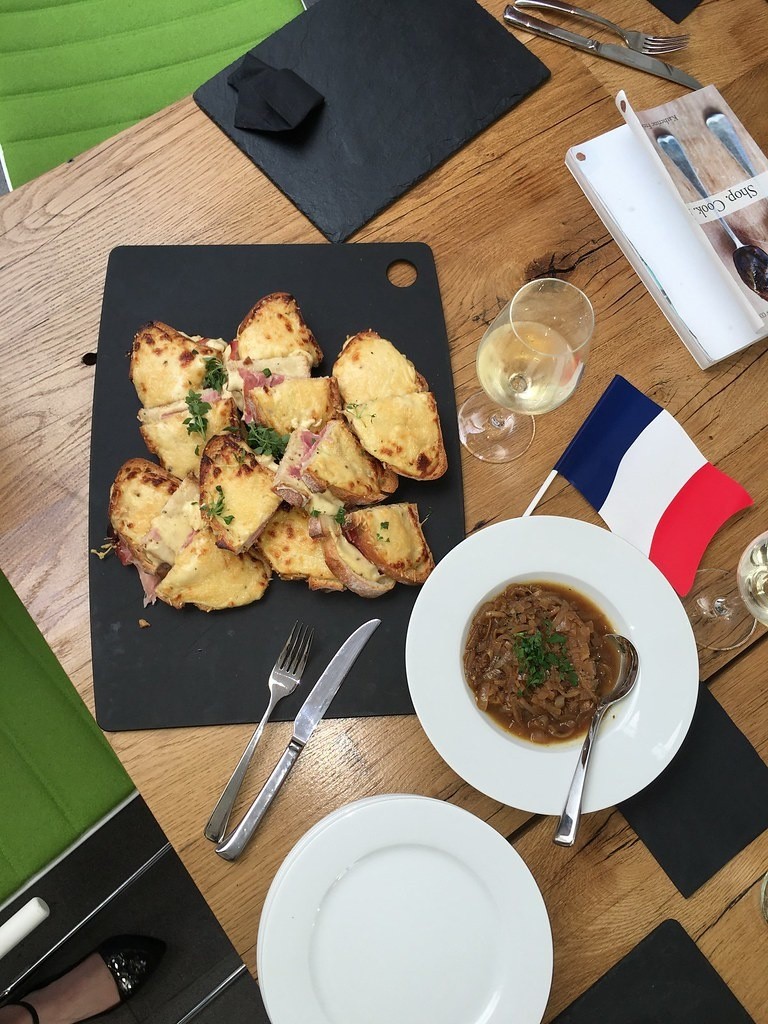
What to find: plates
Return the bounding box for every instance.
[256,794,553,1024]
[404,516,700,816]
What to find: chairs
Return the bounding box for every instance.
[0,572,172,1007]
[0,0,308,194]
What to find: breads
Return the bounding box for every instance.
[105,291,448,611]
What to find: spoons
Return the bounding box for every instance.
[657,134,768,301]
[551,634,639,847]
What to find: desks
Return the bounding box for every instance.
[0,0,768,1024]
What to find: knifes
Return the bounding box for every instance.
[502,5,704,91]
[213,619,383,862]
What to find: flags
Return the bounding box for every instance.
[557,373,756,597]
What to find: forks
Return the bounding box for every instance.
[514,0,690,55]
[203,620,315,843]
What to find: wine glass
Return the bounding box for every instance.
[678,530,768,651]
[457,278,596,464]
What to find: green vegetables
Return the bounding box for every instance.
[512,616,580,697]
